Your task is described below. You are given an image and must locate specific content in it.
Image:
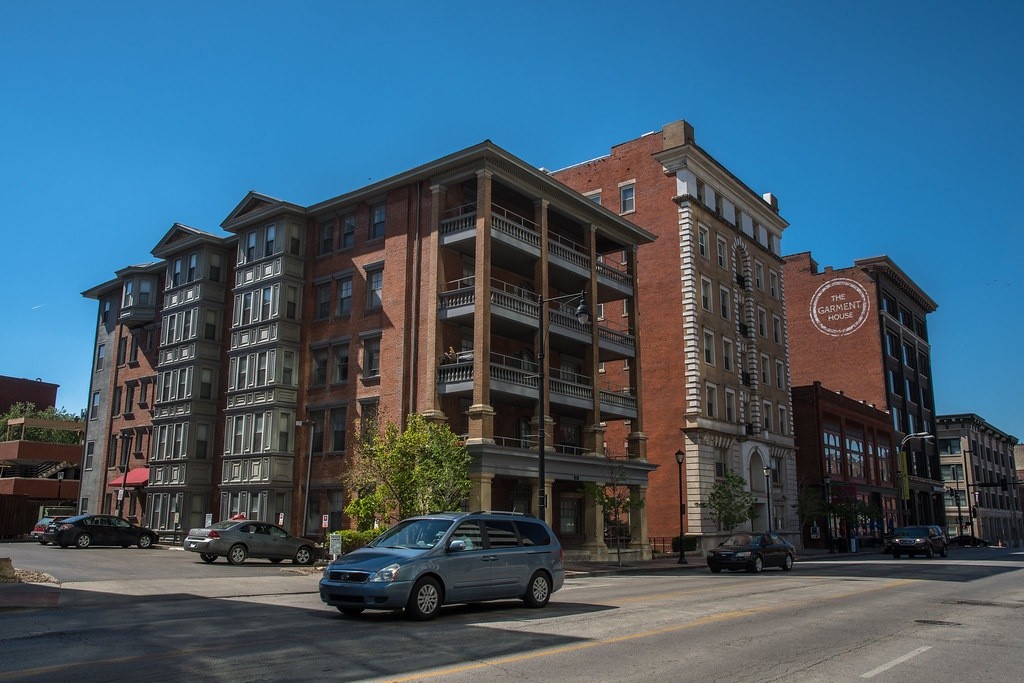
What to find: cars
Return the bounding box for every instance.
[182,518,317,566]
[949,535,990,548]
[706,531,798,575]
[42,514,159,549]
[29,515,79,546]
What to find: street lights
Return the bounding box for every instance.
[963,448,974,536]
[294,418,318,537]
[898,429,936,525]
[535,288,593,519]
[117,434,133,518]
[823,474,836,553]
[762,464,773,532]
[57,471,66,505]
[674,447,690,565]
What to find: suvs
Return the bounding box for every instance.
[318,509,565,619]
[881,524,949,560]
